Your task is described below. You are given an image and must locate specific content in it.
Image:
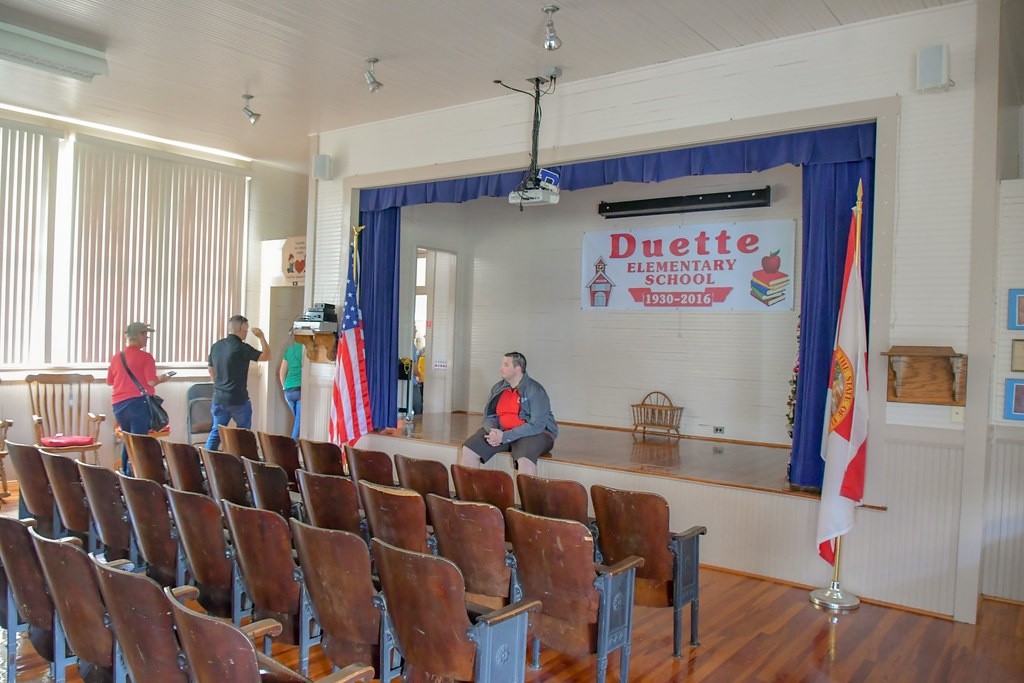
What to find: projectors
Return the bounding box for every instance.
[508,189,560,206]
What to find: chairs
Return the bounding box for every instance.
[187,383,222,463]
[26,373,105,467]
[1,423,708,683]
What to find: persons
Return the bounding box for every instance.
[279,341,303,447]
[107,322,171,477]
[204,315,273,451]
[412,331,426,415]
[460,352,559,503]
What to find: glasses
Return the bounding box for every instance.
[513,352,521,359]
[238,315,246,330]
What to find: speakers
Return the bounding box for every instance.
[313,154,332,182]
[916,45,950,91]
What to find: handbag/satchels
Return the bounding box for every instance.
[144,393,169,432]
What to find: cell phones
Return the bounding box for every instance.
[162,372,177,376]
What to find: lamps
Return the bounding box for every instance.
[542,5,563,50]
[242,94,261,126]
[363,58,383,93]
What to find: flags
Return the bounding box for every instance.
[328,243,373,474]
[818,207,870,567]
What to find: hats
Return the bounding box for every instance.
[126,323,154,336]
